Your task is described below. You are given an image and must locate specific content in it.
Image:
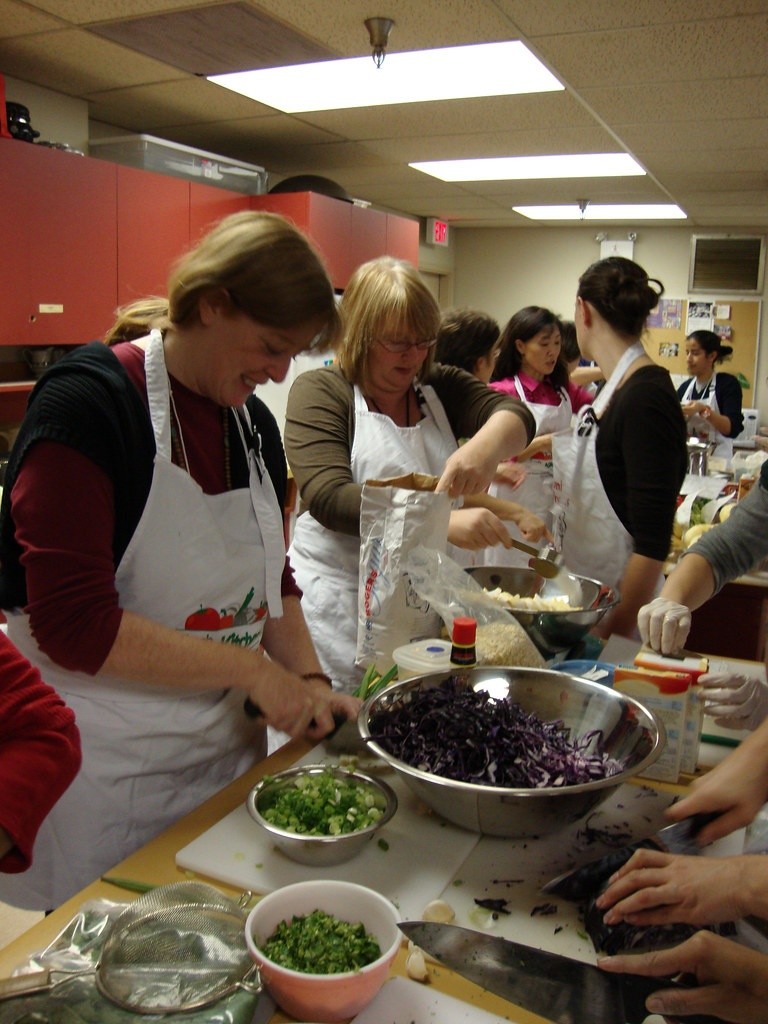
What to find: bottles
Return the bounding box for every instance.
[446,616,479,668]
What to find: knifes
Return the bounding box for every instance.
[395,920,700,1024]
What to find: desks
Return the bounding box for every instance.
[664,560,768,663]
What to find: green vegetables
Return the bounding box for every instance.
[686,497,710,527]
[252,909,382,974]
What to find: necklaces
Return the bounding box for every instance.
[368,390,411,428]
[168,397,233,495]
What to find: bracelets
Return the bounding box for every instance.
[300,671,334,688]
[700,406,711,418]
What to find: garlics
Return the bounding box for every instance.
[405,948,428,982]
[422,900,455,924]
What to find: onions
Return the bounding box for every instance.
[481,587,589,611]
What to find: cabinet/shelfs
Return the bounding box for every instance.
[0,136,420,347]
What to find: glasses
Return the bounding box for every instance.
[377,337,439,354]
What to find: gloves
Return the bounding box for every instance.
[639,599,691,655]
[697,671,768,731]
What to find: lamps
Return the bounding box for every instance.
[595,231,637,261]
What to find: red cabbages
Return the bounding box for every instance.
[361,674,622,790]
[472,813,738,956]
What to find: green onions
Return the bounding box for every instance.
[348,662,399,702]
[259,759,387,853]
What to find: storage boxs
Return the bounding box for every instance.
[613,645,709,785]
[88,134,264,196]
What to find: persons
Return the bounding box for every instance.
[596,703,768,925]
[0,630,83,876]
[637,459,768,731]
[283,256,537,695]
[596,929,768,1024]
[676,330,745,461]
[0,211,363,918]
[435,308,608,570]
[552,257,688,645]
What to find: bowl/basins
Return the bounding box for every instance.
[460,566,622,659]
[245,764,399,866]
[357,664,668,840]
[240,878,403,1024]
[392,638,452,682]
